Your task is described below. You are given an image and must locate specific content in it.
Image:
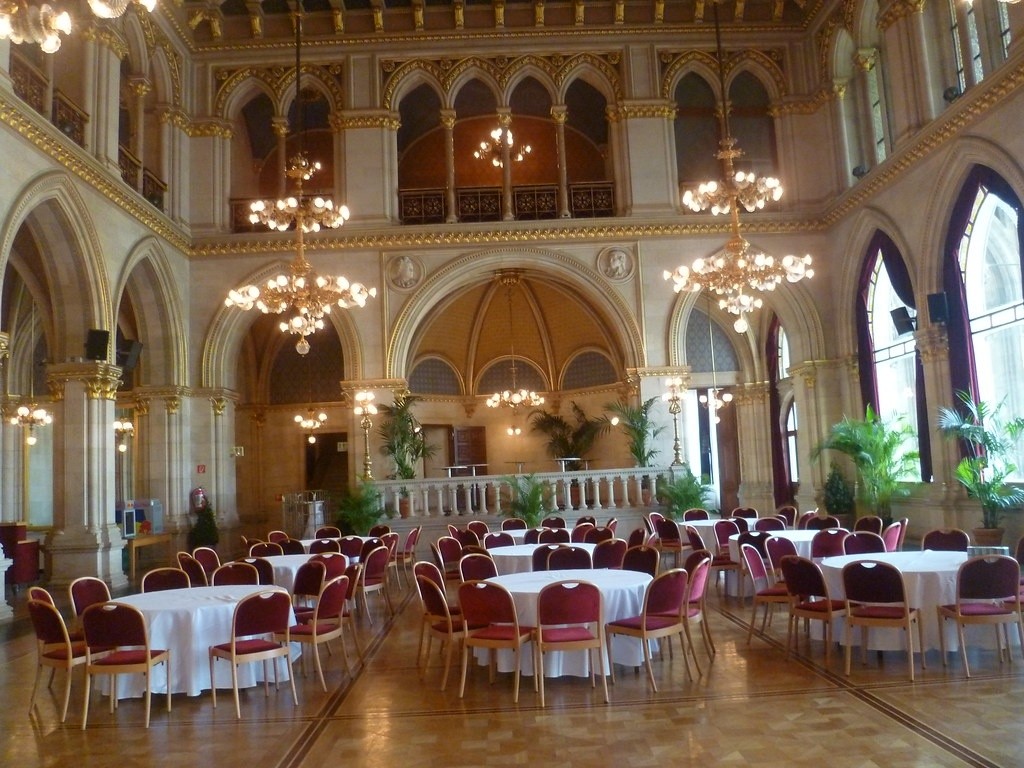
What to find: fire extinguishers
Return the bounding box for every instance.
[191,487,206,513]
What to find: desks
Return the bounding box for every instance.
[810,549,1020,653]
[675,518,762,557]
[123,531,175,580]
[726,527,838,598]
[482,529,575,546]
[296,536,379,555]
[470,569,661,677]
[223,553,357,615]
[486,543,597,574]
[93,585,301,698]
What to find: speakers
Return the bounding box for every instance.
[926,291,950,323]
[890,307,915,336]
[118,339,142,368]
[87,329,109,360]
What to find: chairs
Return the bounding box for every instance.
[1,508,1024,731]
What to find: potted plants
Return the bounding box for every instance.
[603,395,668,506]
[525,399,612,507]
[375,395,442,516]
[812,404,925,530]
[935,387,1024,547]
[819,461,857,528]
[188,500,224,557]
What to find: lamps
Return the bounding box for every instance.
[0,0,814,481]
